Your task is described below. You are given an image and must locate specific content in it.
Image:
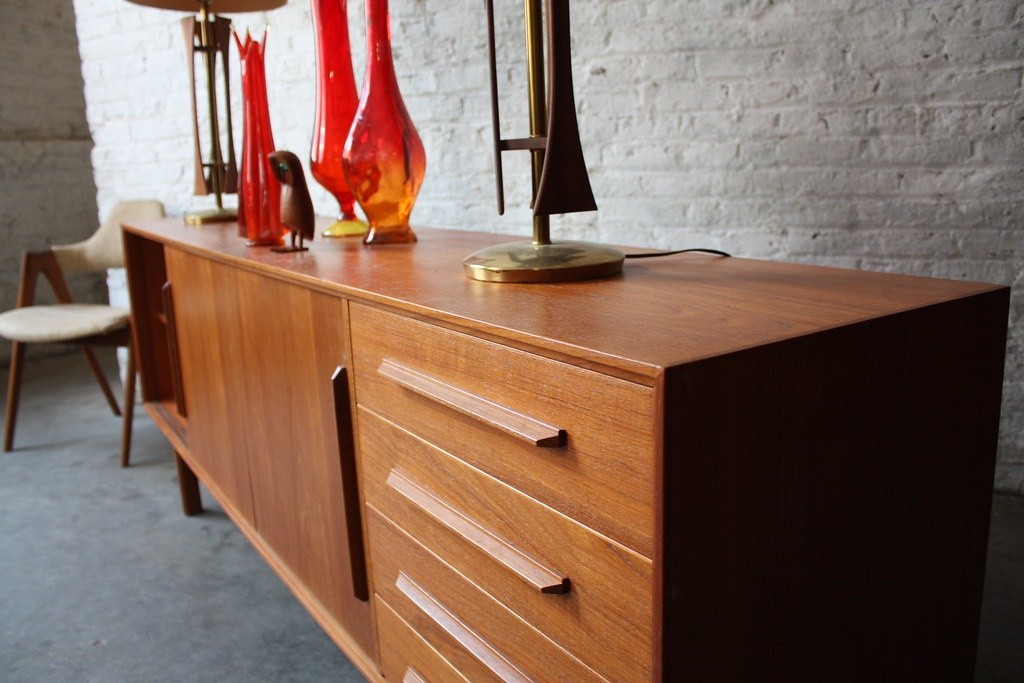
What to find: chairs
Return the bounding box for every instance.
[0,199,165,468]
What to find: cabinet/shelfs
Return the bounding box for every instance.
[121,206,1011,683]
